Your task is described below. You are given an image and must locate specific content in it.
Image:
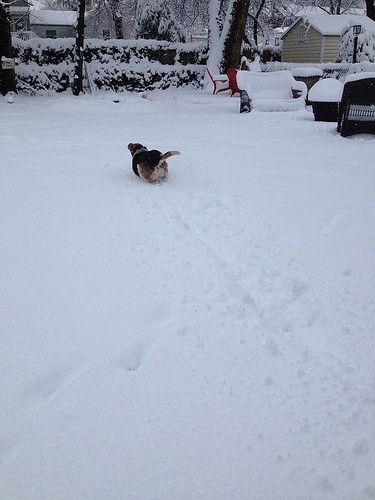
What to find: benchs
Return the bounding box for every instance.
[227,67,309,111]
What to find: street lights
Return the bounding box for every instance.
[351,24,360,62]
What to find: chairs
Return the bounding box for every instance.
[206,68,230,95]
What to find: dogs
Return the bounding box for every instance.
[127,143,182,182]
